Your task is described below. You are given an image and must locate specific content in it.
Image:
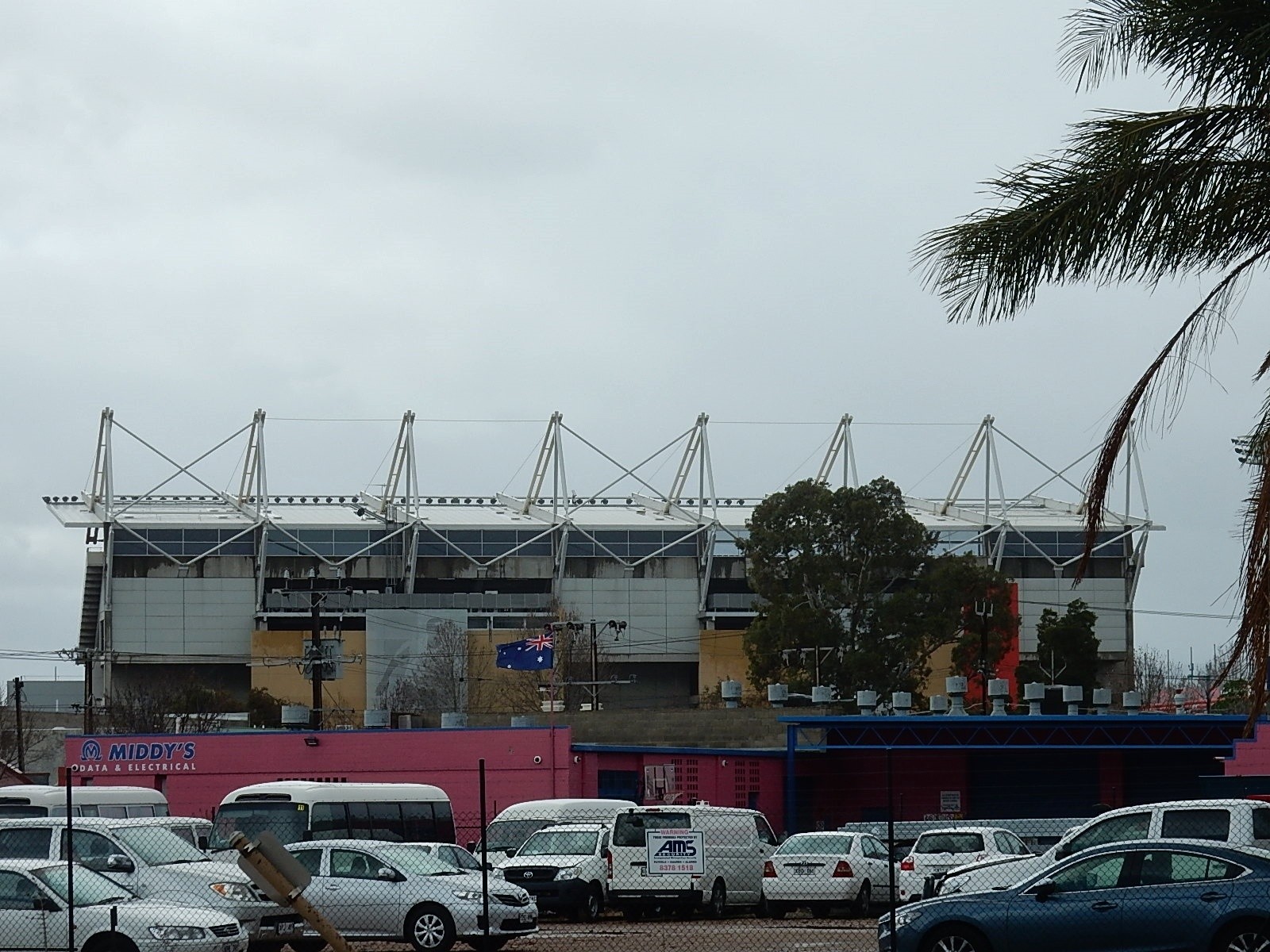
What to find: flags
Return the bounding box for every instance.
[494,632,555,671]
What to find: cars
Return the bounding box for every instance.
[1,858,251,951]
[877,844,1270,952]
[899,825,1032,902]
[761,832,905,919]
[257,838,541,952]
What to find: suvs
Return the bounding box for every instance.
[493,822,614,921]
[938,798,1269,892]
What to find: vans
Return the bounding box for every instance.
[198,780,459,854]
[606,806,787,921]
[0,816,255,944]
[465,799,639,871]
[0,785,170,818]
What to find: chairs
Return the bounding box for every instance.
[930,837,958,851]
[366,856,384,878]
[350,857,368,876]
[89,839,109,871]
